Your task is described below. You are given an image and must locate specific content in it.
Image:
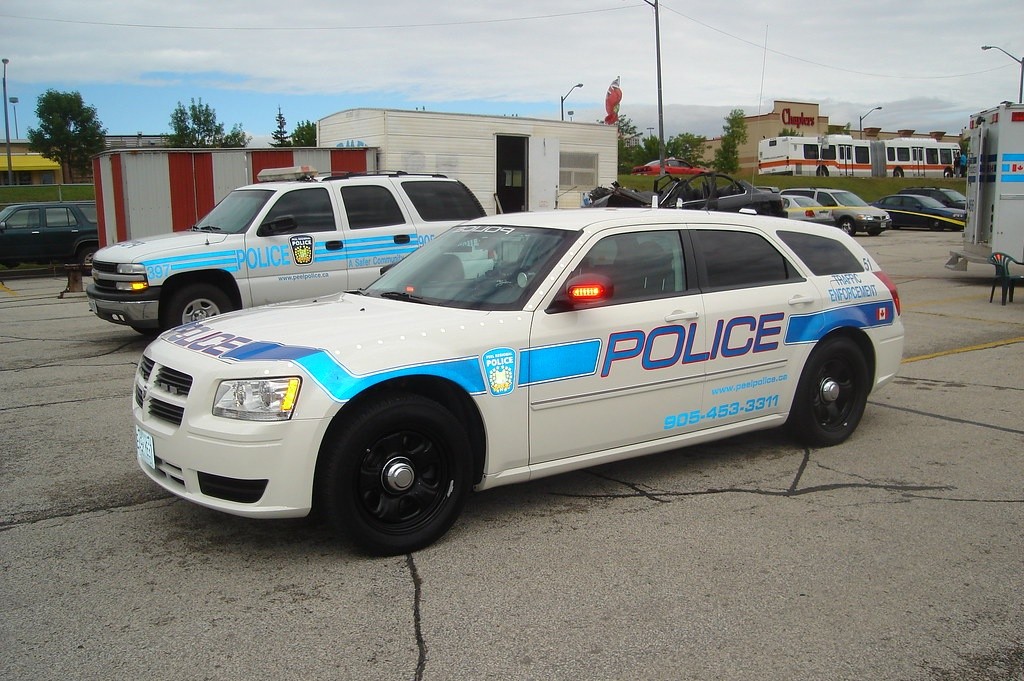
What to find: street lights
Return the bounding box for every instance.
[981,44,1024,105]
[859,106,883,140]
[2,58,14,185]
[9,96,20,139]
[560,83,584,121]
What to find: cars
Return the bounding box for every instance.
[0,200,99,277]
[896,185,966,211]
[132,206,907,555]
[868,195,965,231]
[779,195,836,227]
[631,157,710,176]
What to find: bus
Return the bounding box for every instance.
[756,133,962,179]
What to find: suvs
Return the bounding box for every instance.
[85,165,491,337]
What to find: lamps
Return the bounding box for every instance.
[568,111,574,121]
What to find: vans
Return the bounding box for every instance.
[779,186,893,237]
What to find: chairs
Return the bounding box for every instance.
[987,252,1024,306]
[631,241,670,296]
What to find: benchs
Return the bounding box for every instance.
[63,263,92,292]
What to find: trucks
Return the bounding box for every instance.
[943,102,1023,271]
